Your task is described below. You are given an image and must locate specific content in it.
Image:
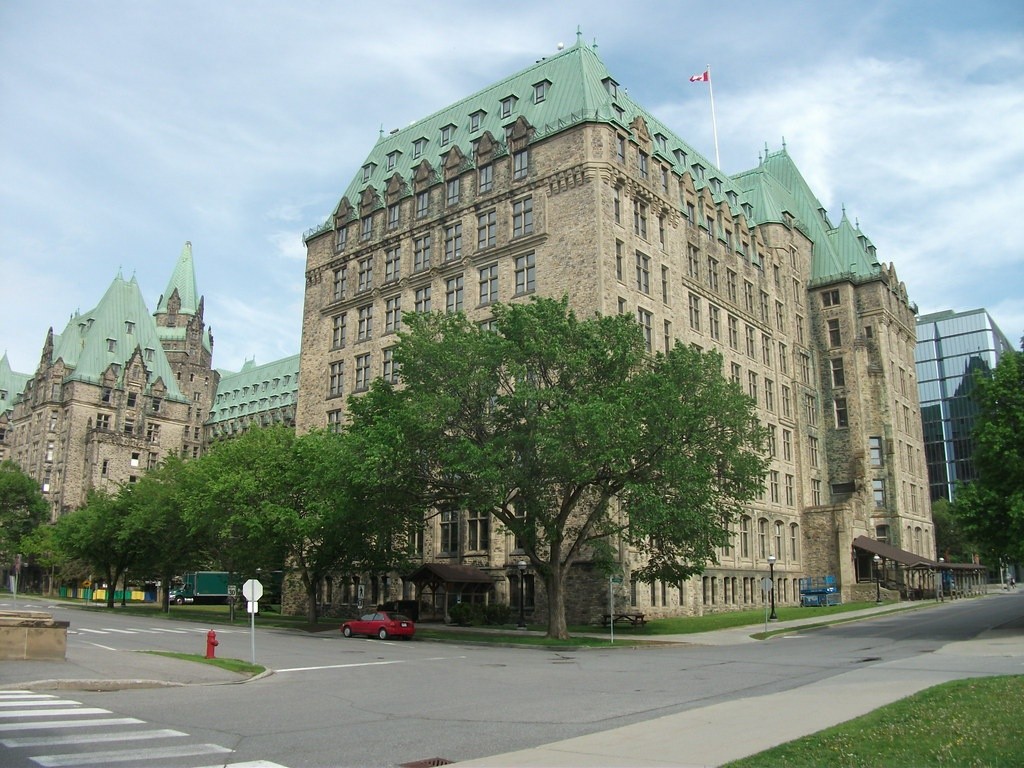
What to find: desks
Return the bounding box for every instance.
[601,612,646,628]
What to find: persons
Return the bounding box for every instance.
[1011,577,1016,588]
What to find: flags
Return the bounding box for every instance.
[690,71,708,82]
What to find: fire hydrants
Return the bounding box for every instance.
[205,629,219,659]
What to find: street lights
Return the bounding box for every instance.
[518,560,527,627]
[939,557,945,595]
[873,554,882,602]
[253,568,262,616]
[768,554,777,619]
[120,567,128,605]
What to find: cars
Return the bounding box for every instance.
[340,611,416,641]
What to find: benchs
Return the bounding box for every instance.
[599,619,650,628]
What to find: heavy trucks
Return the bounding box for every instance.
[168,569,282,605]
